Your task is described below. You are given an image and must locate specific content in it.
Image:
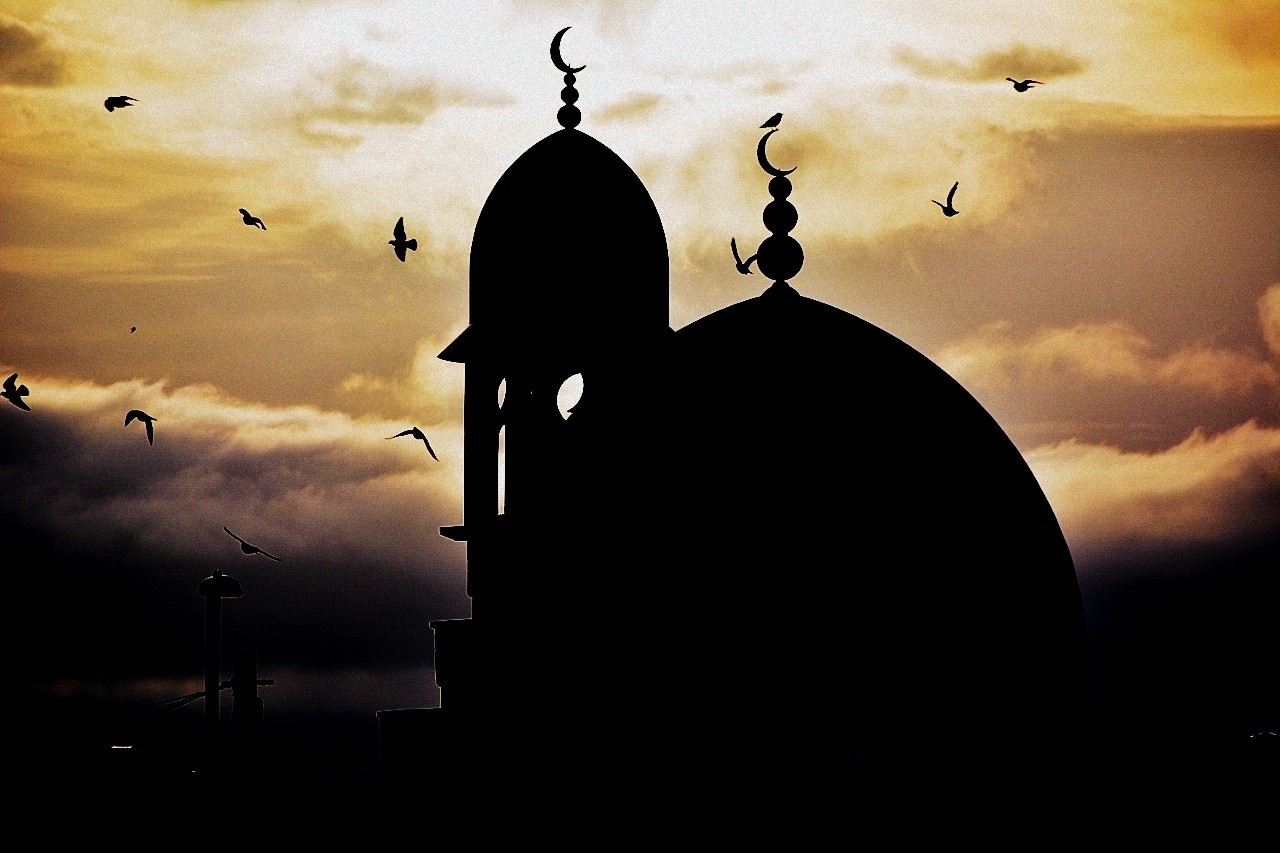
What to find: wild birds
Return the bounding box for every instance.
[385,426,439,461]
[388,217,418,262]
[130,326,136,333]
[0,373,32,412]
[239,209,267,231]
[931,181,960,217]
[1006,78,1045,92]
[224,526,283,561]
[124,409,157,446]
[104,96,138,112]
[759,113,784,130]
[731,238,757,276]
